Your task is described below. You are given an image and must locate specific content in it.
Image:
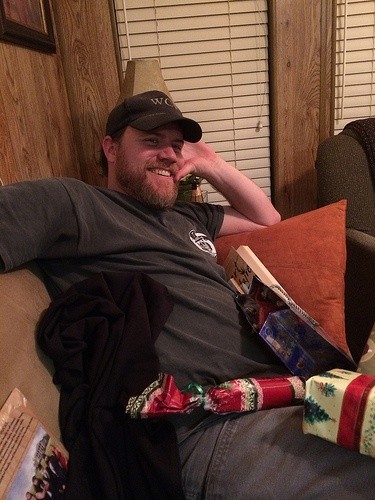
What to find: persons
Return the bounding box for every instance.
[0,90,374,499]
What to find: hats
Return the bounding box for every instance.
[105,90,202,144]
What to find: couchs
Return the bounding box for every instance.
[316,116,374,333]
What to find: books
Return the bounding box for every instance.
[223,245,294,302]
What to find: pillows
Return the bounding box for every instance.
[211,196,356,372]
